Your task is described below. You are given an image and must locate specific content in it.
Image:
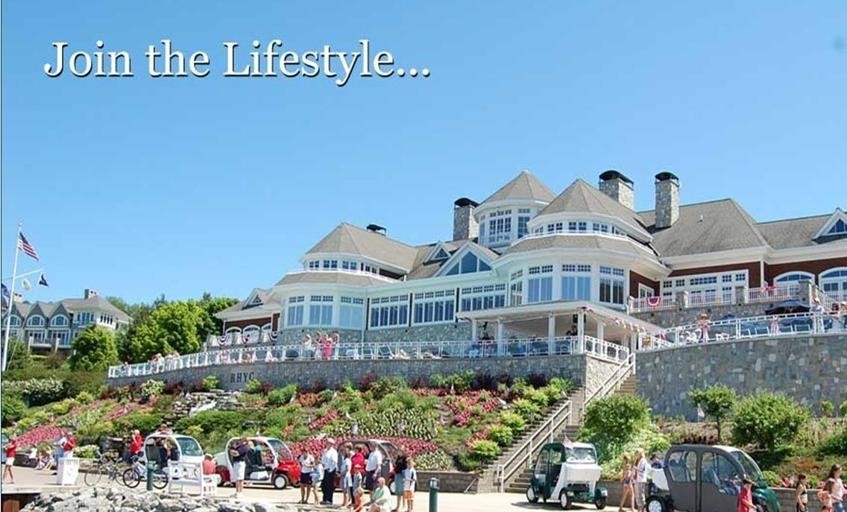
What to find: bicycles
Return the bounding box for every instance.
[122,452,168,489]
[83,450,136,487]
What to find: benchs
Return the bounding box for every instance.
[165,457,222,499]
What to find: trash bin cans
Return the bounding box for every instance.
[56,457,81,486]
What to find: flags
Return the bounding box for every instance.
[18,230,38,260]
[39,276,47,285]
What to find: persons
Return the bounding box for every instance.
[297,437,415,512]
[60,431,75,457]
[321,333,332,360]
[737,478,757,512]
[829,302,839,330]
[54,430,66,468]
[2,435,15,484]
[809,298,823,332]
[229,437,247,493]
[202,453,215,489]
[827,464,846,512]
[124,361,128,365]
[147,350,182,373]
[697,313,710,343]
[793,473,808,511]
[840,301,846,329]
[818,477,834,512]
[128,423,179,473]
[303,334,311,360]
[618,449,664,512]
[313,331,322,360]
